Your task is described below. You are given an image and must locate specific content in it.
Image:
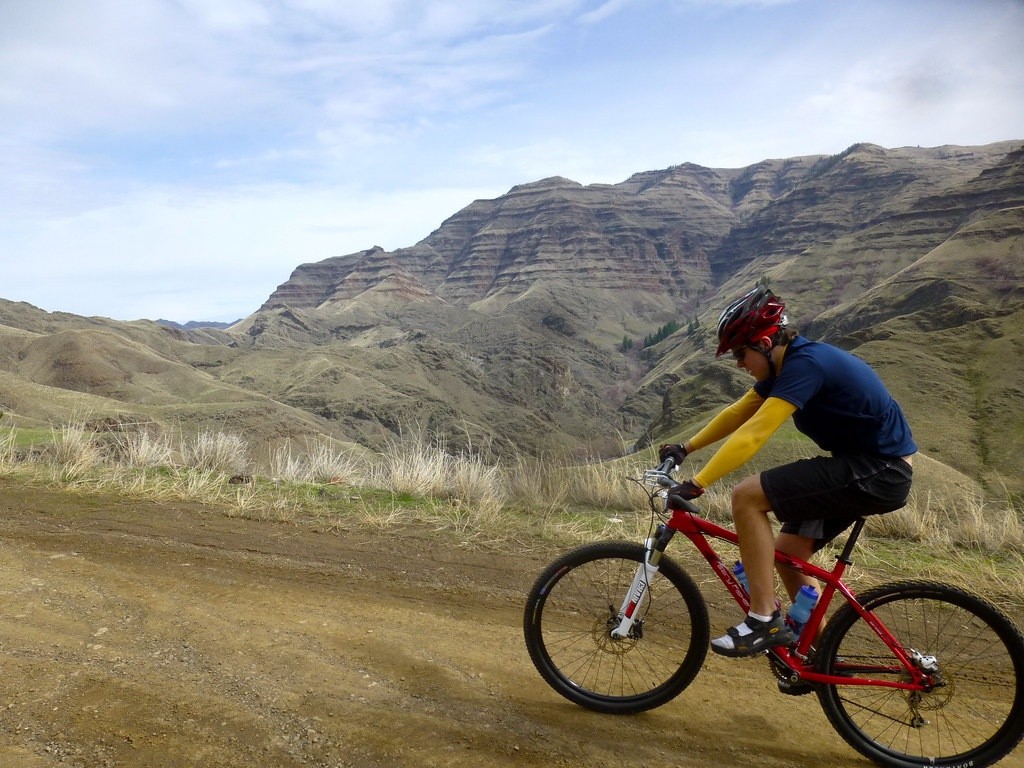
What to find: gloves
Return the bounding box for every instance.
[658,442,688,468]
[666,479,705,513]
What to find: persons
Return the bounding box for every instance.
[658,285,918,694]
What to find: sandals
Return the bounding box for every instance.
[777,653,854,696]
[710,608,794,657]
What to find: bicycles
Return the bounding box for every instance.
[523,450,1024,767]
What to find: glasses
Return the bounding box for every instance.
[732,339,760,360]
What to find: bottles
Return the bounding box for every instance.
[782,585,820,643]
[733,559,750,594]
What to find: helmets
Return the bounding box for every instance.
[714,284,785,359]
